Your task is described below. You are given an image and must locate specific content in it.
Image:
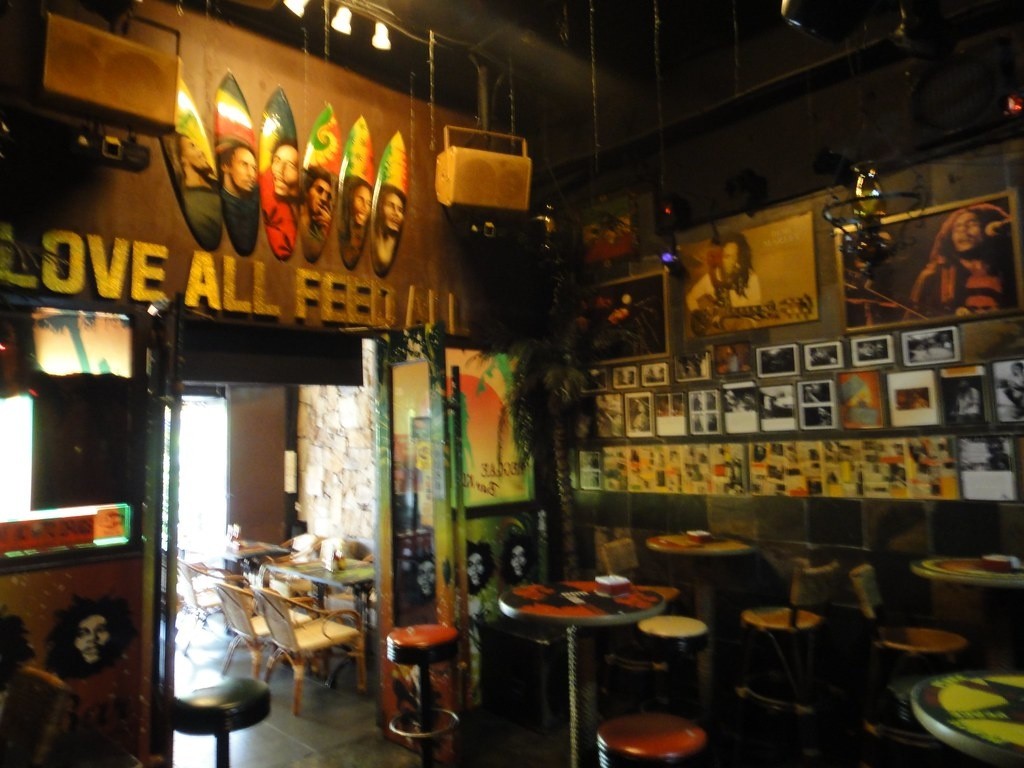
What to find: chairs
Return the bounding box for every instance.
[602,538,647,585]
[740,558,843,713]
[171,557,368,716]
[847,563,969,745]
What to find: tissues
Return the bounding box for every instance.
[687,531,711,544]
[595,575,631,598]
[982,555,1020,571]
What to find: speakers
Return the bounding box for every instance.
[21,11,177,138]
[435,146,532,209]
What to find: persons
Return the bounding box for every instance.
[448,535,495,598]
[900,203,1021,322]
[162,129,416,272]
[687,230,765,335]
[499,531,536,587]
[47,596,139,678]
[406,547,452,601]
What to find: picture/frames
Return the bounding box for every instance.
[834,187,1023,336]
[584,264,671,366]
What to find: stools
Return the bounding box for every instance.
[637,616,708,719]
[174,675,272,768]
[385,623,459,768]
[592,712,709,768]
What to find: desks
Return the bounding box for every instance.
[910,554,1024,768]
[646,534,754,688]
[260,560,377,690]
[495,579,684,768]
